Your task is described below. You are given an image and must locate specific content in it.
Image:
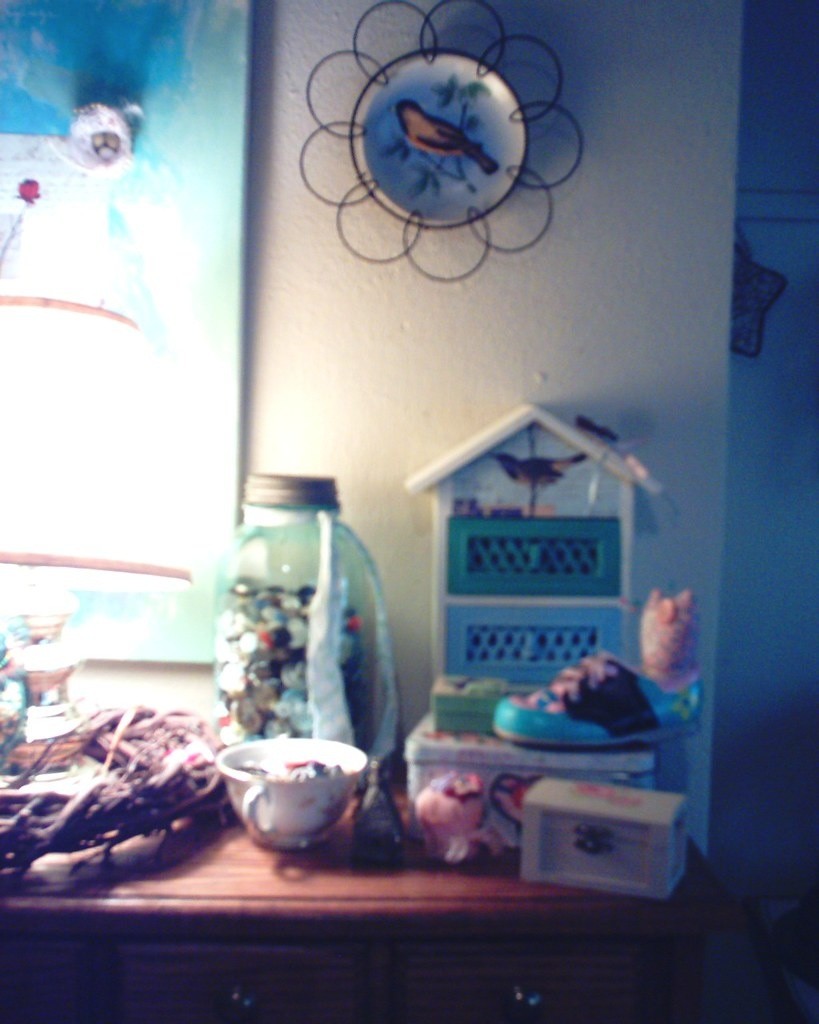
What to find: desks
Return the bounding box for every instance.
[0,763,741,1024]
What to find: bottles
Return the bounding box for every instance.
[216,471,398,791]
[5,601,80,782]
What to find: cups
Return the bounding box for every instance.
[214,738,369,851]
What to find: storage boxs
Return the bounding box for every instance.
[403,711,655,847]
